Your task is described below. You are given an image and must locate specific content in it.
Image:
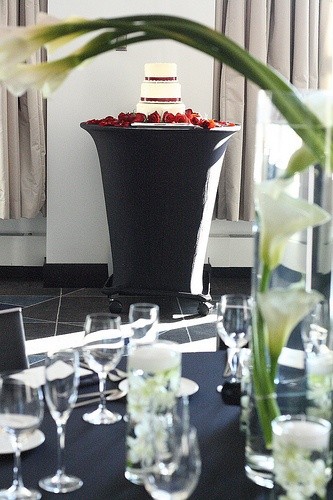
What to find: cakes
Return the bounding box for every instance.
[135,62,185,120]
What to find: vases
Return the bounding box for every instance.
[243,90,333,488]
[80,119,241,296]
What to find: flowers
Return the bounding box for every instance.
[0,11,333,450]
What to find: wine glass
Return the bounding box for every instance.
[82,316,125,425]
[0,370,44,500]
[39,349,83,494]
[217,294,252,391]
[299,302,330,389]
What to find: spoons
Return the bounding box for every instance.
[74,390,127,407]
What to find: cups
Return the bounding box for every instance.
[270,416,332,500]
[145,427,204,500]
[123,342,182,485]
[129,302,160,345]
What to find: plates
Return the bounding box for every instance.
[119,378,199,397]
